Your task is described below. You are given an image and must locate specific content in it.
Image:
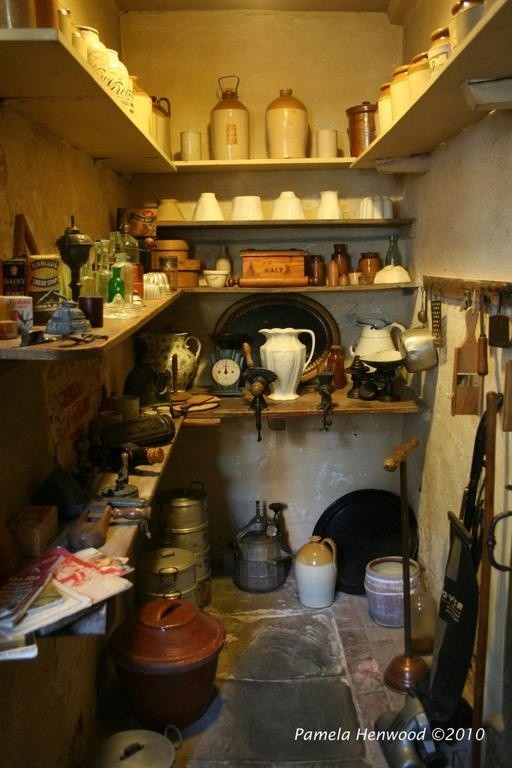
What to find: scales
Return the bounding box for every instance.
[207,330,245,397]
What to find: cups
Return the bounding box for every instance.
[180,132,201,161]
[315,129,338,159]
[319,190,339,219]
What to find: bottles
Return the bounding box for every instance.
[96,252,111,302]
[309,255,325,285]
[215,244,231,273]
[111,253,133,303]
[385,233,402,267]
[327,260,339,286]
[159,255,178,291]
[80,261,95,298]
[107,266,125,303]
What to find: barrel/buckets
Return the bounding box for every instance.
[364,555,422,629]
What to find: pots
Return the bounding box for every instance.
[157,480,210,609]
[89,723,183,767]
[399,288,438,373]
[139,548,199,609]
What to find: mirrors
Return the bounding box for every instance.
[214,292,340,385]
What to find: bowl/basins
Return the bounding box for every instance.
[270,191,306,220]
[154,199,185,221]
[230,196,263,220]
[203,270,230,289]
[192,192,225,221]
